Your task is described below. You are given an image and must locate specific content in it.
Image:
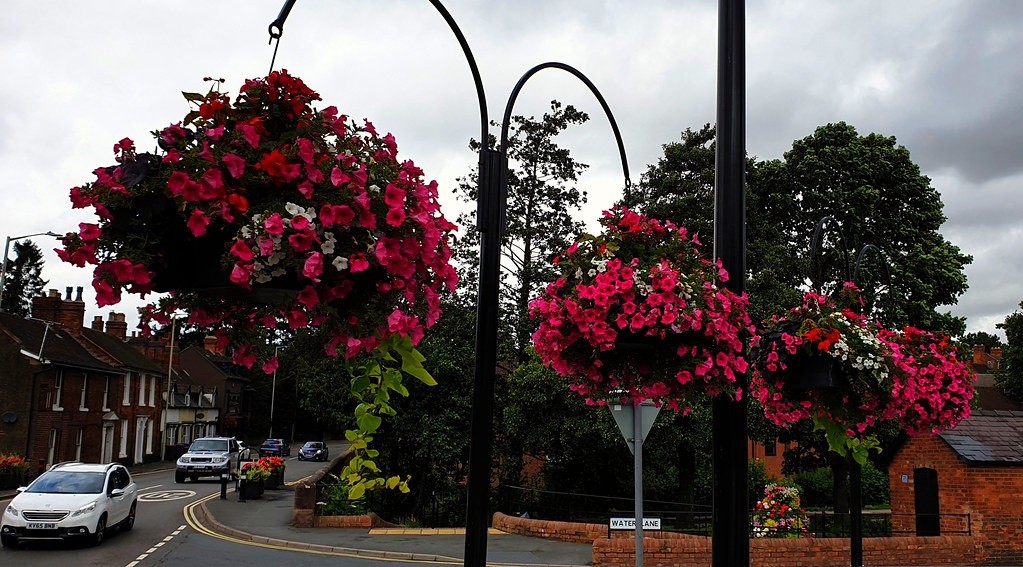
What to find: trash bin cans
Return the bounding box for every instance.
[165,444,185,461]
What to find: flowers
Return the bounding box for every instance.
[527,206,759,418]
[750,481,816,540]
[44,66,460,503]
[748,281,979,465]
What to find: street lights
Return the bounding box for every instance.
[0,232,64,299]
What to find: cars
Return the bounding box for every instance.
[237,439,252,461]
[298,442,329,462]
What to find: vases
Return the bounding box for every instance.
[240,481,265,499]
[265,469,285,490]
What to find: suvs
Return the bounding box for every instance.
[0,460,138,550]
[259,438,291,457]
[174,435,243,482]
